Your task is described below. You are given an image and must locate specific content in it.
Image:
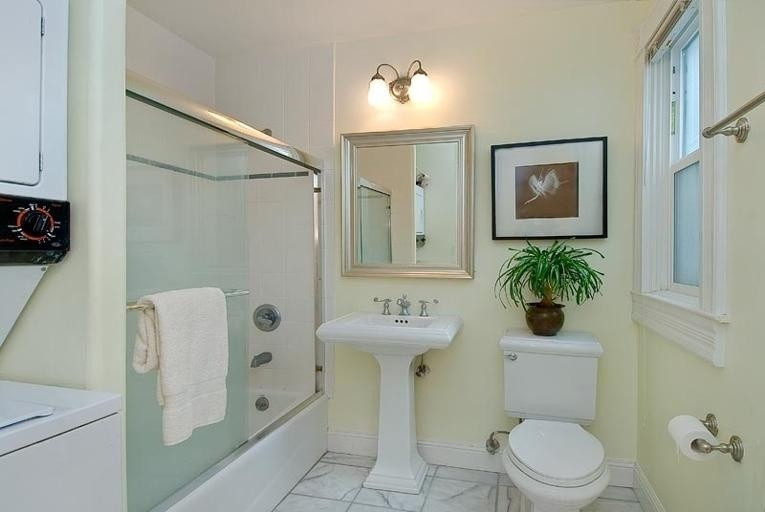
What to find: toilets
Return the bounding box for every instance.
[497,326,612,512]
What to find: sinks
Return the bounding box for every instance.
[314,310,464,358]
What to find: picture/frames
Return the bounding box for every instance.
[490,136,608,240]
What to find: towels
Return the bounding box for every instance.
[133,287,229,447]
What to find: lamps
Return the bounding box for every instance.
[367,58,433,108]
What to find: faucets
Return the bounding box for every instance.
[396,298,411,316]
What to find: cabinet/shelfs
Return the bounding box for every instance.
[0,396,125,512]
[415,184,425,236]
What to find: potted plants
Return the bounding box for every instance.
[493,234,607,335]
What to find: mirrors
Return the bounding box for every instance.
[339,123,475,279]
